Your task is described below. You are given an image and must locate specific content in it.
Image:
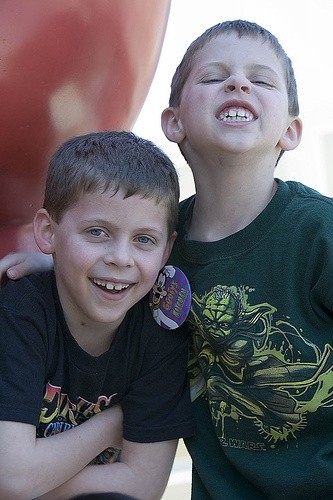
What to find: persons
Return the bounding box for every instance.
[1,21,333,500]
[1,132,189,500]
